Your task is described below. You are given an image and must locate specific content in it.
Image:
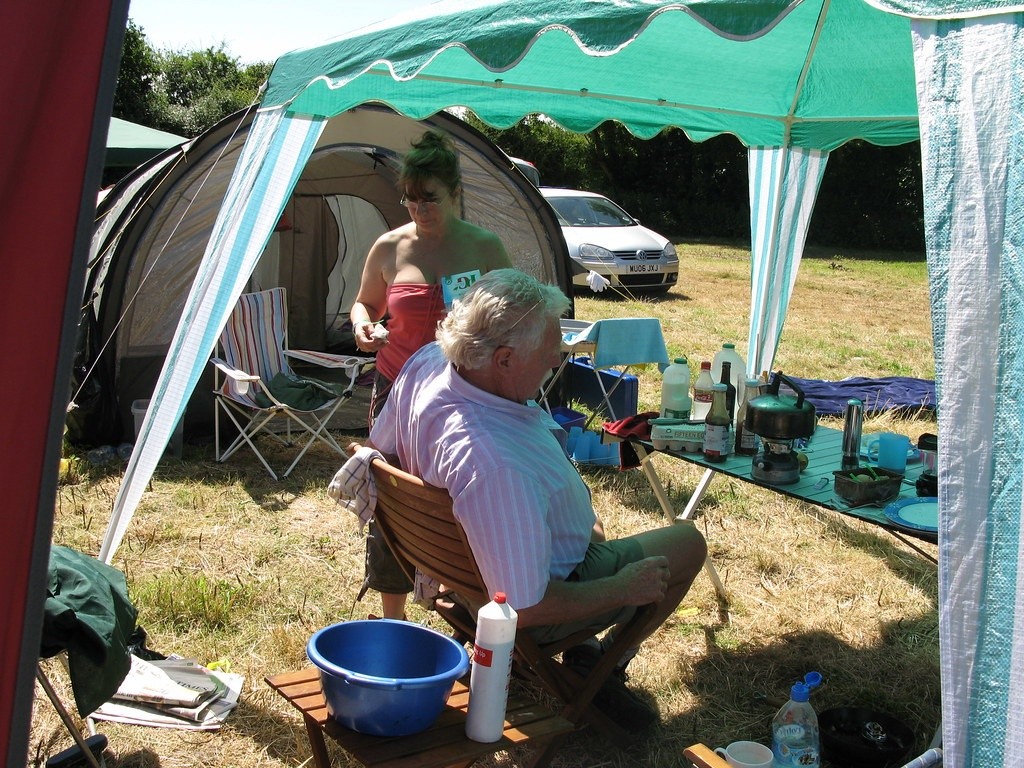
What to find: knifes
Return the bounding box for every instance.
[647,419,705,424]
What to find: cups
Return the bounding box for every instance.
[713,741,774,768]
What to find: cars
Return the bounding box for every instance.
[537,186,680,297]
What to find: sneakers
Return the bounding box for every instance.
[560,644,656,730]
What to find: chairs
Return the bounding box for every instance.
[29,548,141,768]
[345,442,665,768]
[208,286,374,480]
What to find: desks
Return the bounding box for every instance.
[604,402,946,594]
[264,668,571,768]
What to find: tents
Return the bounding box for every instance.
[82,84,578,445]
[98,0,929,563]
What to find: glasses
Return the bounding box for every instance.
[506,276,548,333]
[399,184,455,209]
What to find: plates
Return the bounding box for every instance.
[859,442,921,461]
[882,496,938,532]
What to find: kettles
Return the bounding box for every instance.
[745,370,819,438]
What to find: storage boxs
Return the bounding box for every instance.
[130,397,190,460]
[552,405,589,432]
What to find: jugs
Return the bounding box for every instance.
[867,432,910,474]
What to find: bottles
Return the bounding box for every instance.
[701,384,731,463]
[659,358,692,420]
[464,591,519,743]
[711,343,747,426]
[719,361,736,428]
[771,671,820,768]
[87,442,134,462]
[692,360,715,420]
[734,378,761,457]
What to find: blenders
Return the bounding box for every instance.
[914,432,939,497]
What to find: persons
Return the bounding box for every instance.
[344,124,518,623]
[369,268,706,734]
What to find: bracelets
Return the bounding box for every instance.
[353,320,370,336]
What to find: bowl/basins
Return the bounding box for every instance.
[818,706,917,768]
[305,617,471,737]
